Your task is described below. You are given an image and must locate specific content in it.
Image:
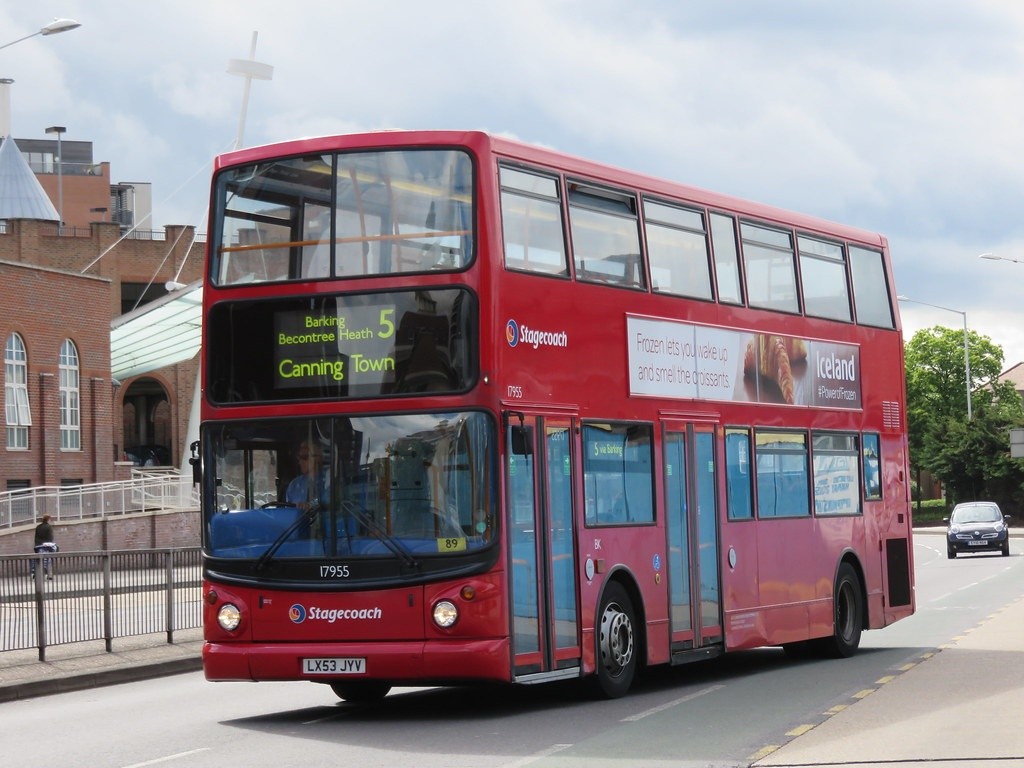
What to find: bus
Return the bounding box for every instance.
[189,126,914,713]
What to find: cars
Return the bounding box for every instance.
[942,501,1014,558]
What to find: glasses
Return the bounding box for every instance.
[296,455,320,460]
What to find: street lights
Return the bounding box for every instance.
[895,295,972,424]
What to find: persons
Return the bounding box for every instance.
[283,439,348,537]
[32,513,55,580]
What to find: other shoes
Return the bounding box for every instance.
[47,578,54,581]
